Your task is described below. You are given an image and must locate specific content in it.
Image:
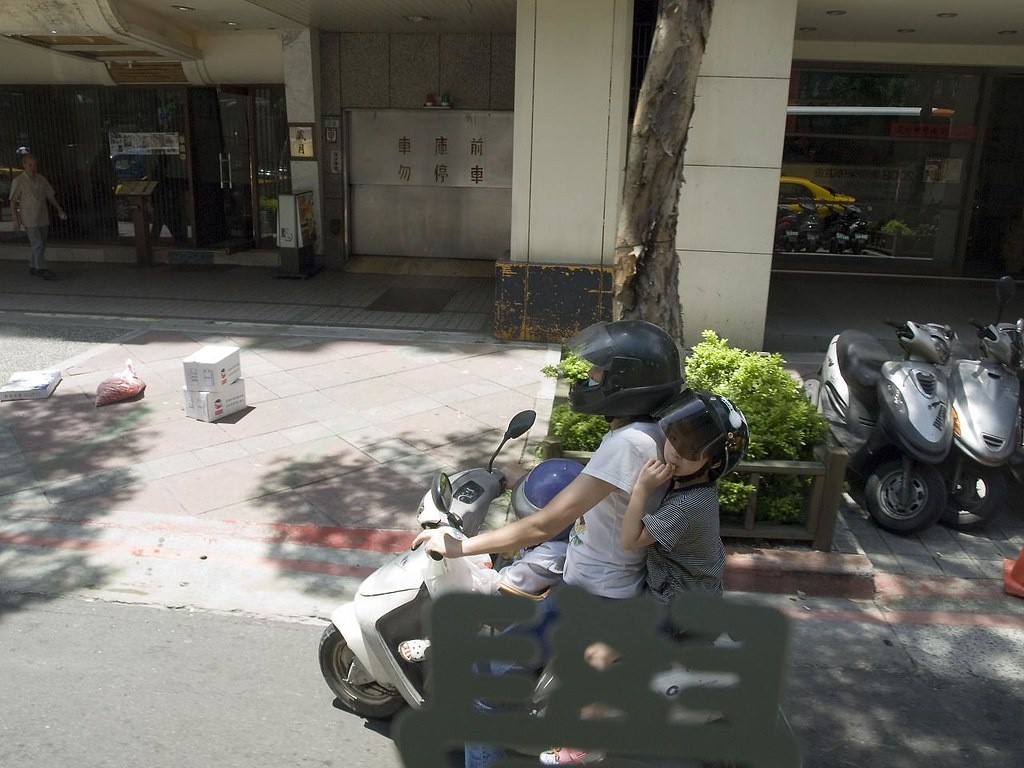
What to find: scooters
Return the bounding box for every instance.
[318,409,748,767]
[936,275,1024,534]
[774,195,872,255]
[799,316,958,537]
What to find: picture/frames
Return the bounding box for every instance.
[287,120,316,163]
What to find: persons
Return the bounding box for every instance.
[411,320,749,768]
[9,153,68,279]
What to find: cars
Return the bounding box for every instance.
[777,176,856,219]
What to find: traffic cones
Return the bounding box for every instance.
[1002,542,1023,599]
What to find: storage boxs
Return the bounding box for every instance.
[181,378,246,424]
[0,370,63,402]
[182,345,243,394]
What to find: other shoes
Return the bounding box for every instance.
[30,268,39,277]
[39,269,56,278]
[539,745,607,767]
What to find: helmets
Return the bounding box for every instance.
[650,388,749,483]
[511,459,585,539]
[568,319,684,417]
